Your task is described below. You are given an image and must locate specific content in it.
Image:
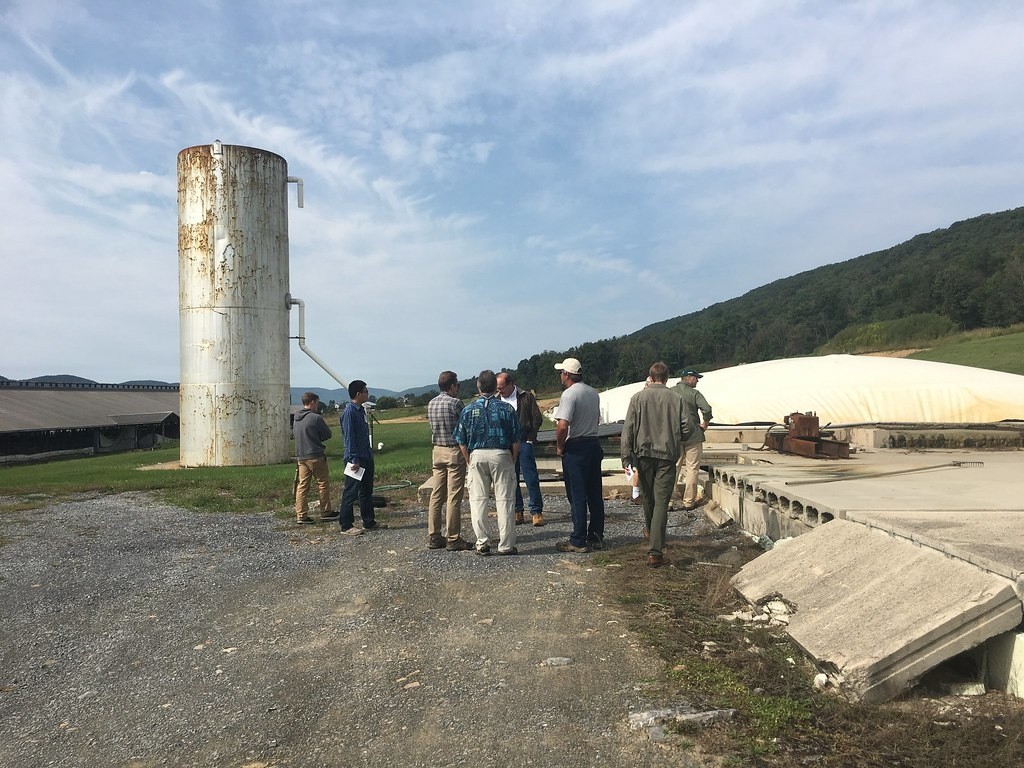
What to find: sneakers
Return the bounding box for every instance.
[320,510,341,520]
[556,540,588,553]
[648,556,671,568]
[296,517,315,524]
[628,495,645,507]
[585,536,603,549]
[642,526,651,542]
[428,535,447,548]
[444,536,474,551]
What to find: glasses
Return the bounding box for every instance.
[453,383,460,389]
[558,370,566,375]
[495,383,509,391]
[361,390,369,394]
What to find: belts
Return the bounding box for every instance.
[566,435,594,442]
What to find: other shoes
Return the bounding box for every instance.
[514,512,525,524]
[530,512,545,526]
[361,523,389,532]
[666,505,676,512]
[473,546,491,556]
[686,502,698,510]
[496,547,518,554]
[340,525,364,536]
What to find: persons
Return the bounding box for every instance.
[339,380,388,536]
[556,357,604,553]
[667,368,713,512]
[630,374,652,507]
[494,373,543,526]
[293,392,340,524]
[428,371,474,550]
[621,362,693,568]
[451,370,523,558]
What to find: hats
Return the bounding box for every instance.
[553,358,584,375]
[679,369,703,378]
